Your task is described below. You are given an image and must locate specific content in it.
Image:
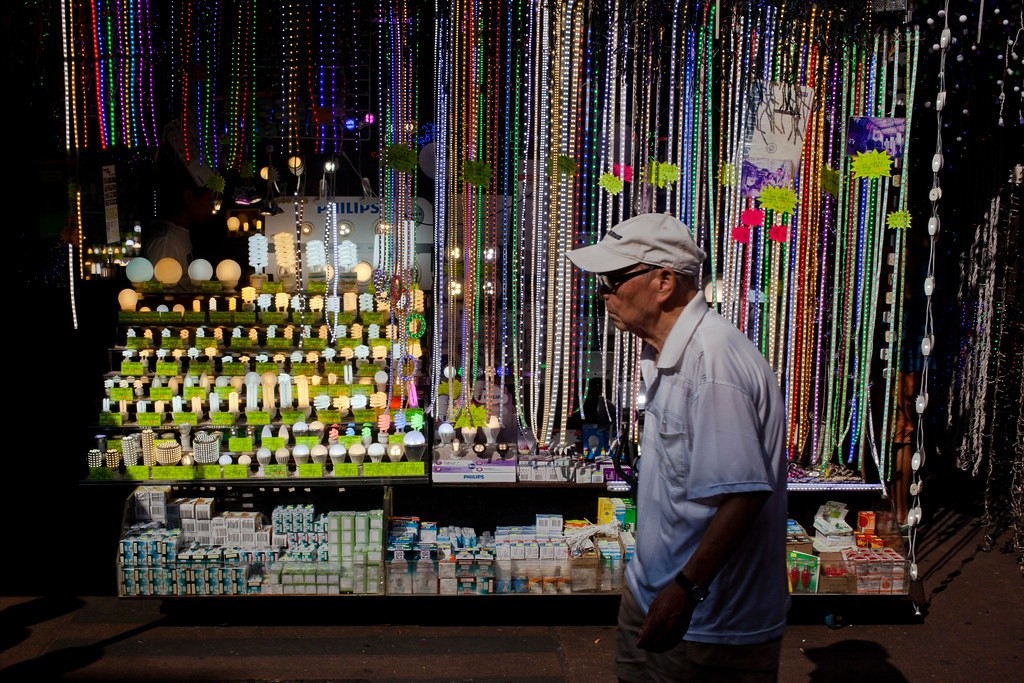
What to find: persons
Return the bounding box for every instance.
[144,168,220,280]
[564,213,790,683]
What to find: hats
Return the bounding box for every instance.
[562,213,708,276]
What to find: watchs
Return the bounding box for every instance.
[674,571,712,603]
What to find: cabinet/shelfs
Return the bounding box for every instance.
[117,462,913,596]
[80,274,430,485]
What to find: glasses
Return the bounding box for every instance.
[595,266,681,291]
